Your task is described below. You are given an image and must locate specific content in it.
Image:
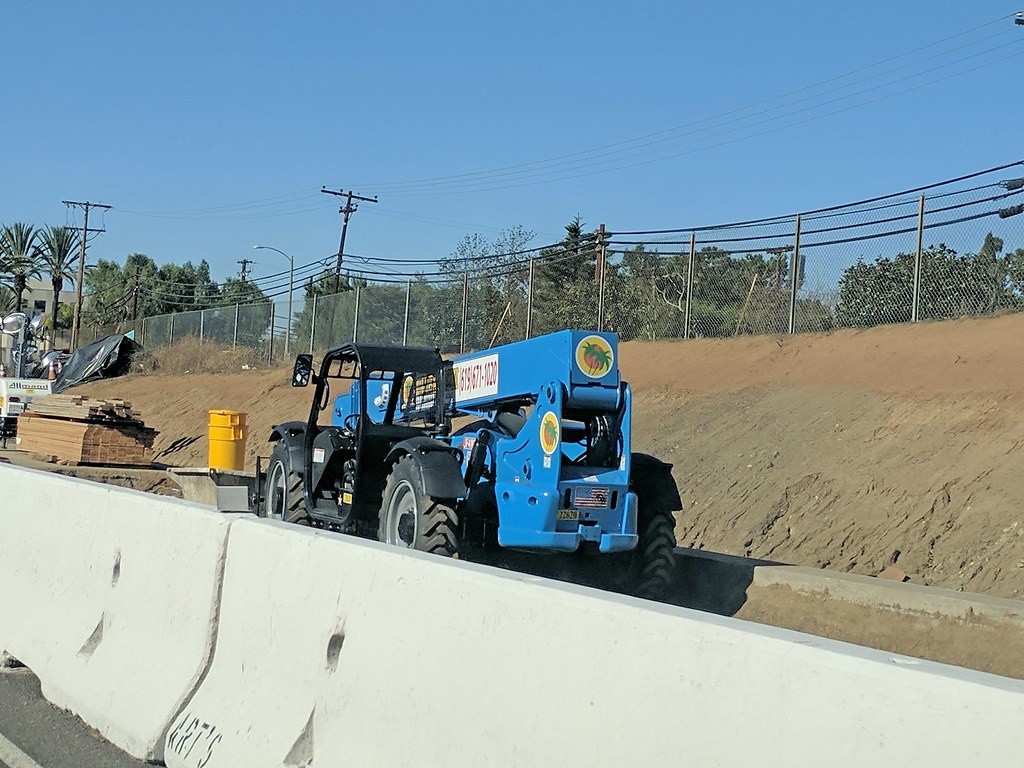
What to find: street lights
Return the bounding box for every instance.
[254,245,295,361]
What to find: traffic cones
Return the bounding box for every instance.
[1,364,6,377]
[57,360,62,373]
[48,361,55,381]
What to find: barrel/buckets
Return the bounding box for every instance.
[208,408,249,471]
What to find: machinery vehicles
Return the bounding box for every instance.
[249,331,685,604]
